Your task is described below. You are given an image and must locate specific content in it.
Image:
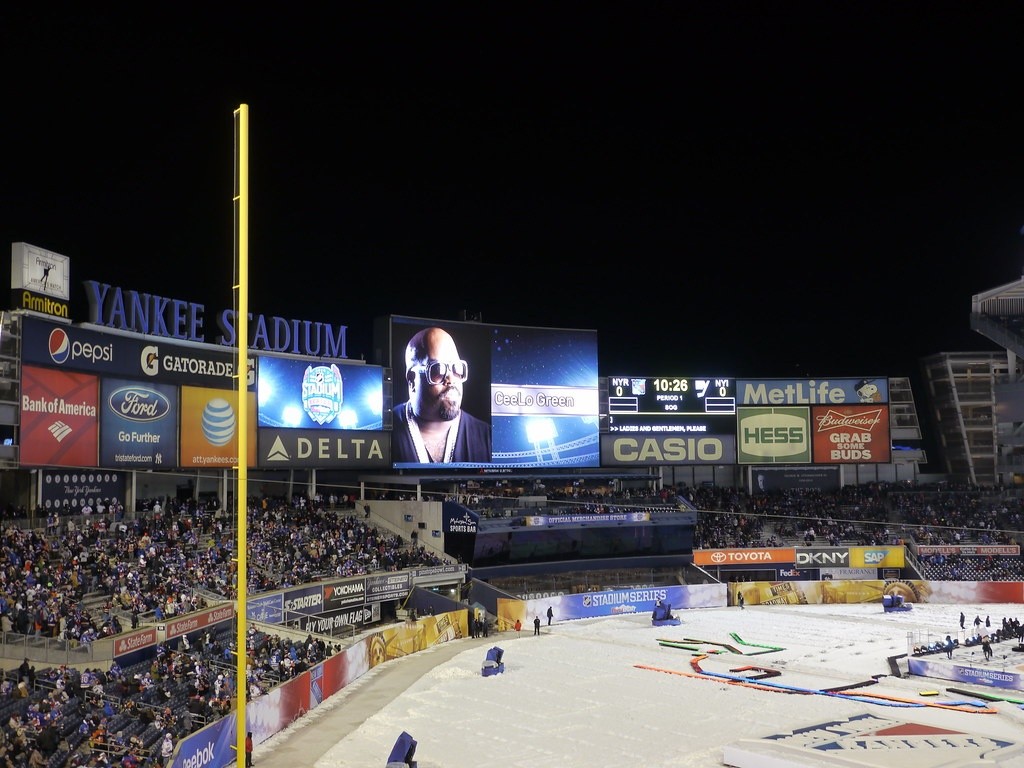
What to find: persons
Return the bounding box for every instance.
[842,480,1024,580]
[982,635,993,661]
[409,611,417,621]
[514,620,521,638]
[960,612,965,629]
[428,605,433,615]
[946,635,953,659]
[986,616,991,633]
[471,617,488,638]
[548,607,553,625]
[534,616,540,635]
[755,474,772,493]
[655,598,661,608]
[1002,617,1024,642]
[974,616,983,632]
[398,328,492,463]
[740,595,744,609]
[0,495,450,768]
[453,485,857,546]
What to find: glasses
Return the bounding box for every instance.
[410,359,469,386]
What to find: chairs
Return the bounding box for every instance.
[0,490,462,768]
[913,627,1023,655]
[445,474,1024,600]
